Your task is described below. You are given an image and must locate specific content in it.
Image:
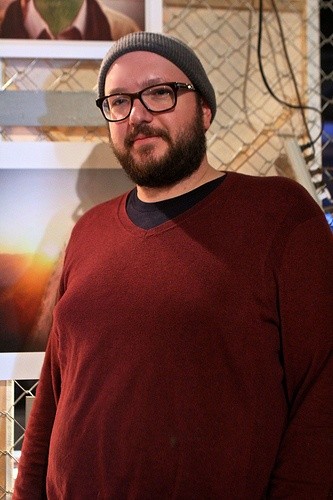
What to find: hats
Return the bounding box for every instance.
[98,31,217,131]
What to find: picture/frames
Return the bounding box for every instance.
[0,0,163,59]
[0,142,136,380]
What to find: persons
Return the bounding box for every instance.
[10,30,333,499]
[0,1,145,40]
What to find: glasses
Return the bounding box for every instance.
[95,82,197,122]
